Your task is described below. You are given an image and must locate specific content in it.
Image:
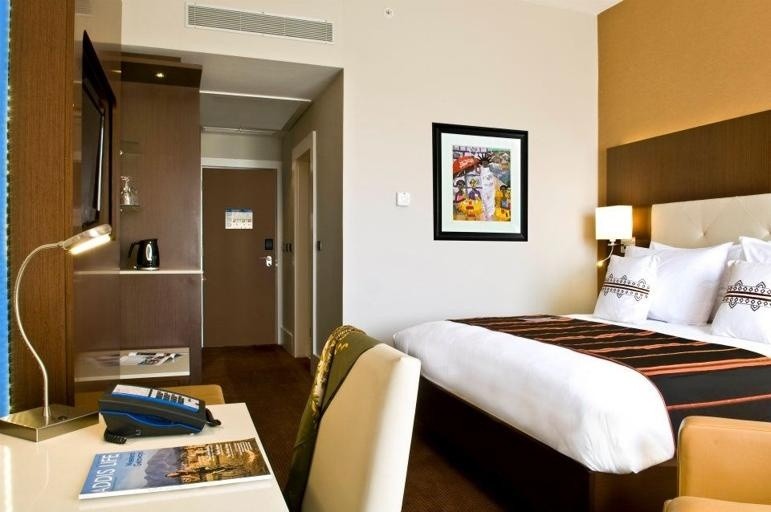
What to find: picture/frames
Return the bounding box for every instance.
[432,122,529,242]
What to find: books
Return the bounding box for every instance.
[121,351,181,366]
[77,437,270,499]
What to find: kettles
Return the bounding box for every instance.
[127,237,161,270]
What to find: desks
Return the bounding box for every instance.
[0,401,291,511]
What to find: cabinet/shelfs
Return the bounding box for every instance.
[63,263,203,382]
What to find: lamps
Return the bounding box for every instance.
[594,205,636,268]
[0,223,115,442]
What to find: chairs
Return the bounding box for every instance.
[288,325,422,512]
[666,414,770,511]
[592,237,771,344]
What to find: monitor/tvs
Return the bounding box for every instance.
[81,73,105,224]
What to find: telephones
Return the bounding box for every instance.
[97,383,206,436]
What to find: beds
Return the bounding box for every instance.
[391,193,771,510]
